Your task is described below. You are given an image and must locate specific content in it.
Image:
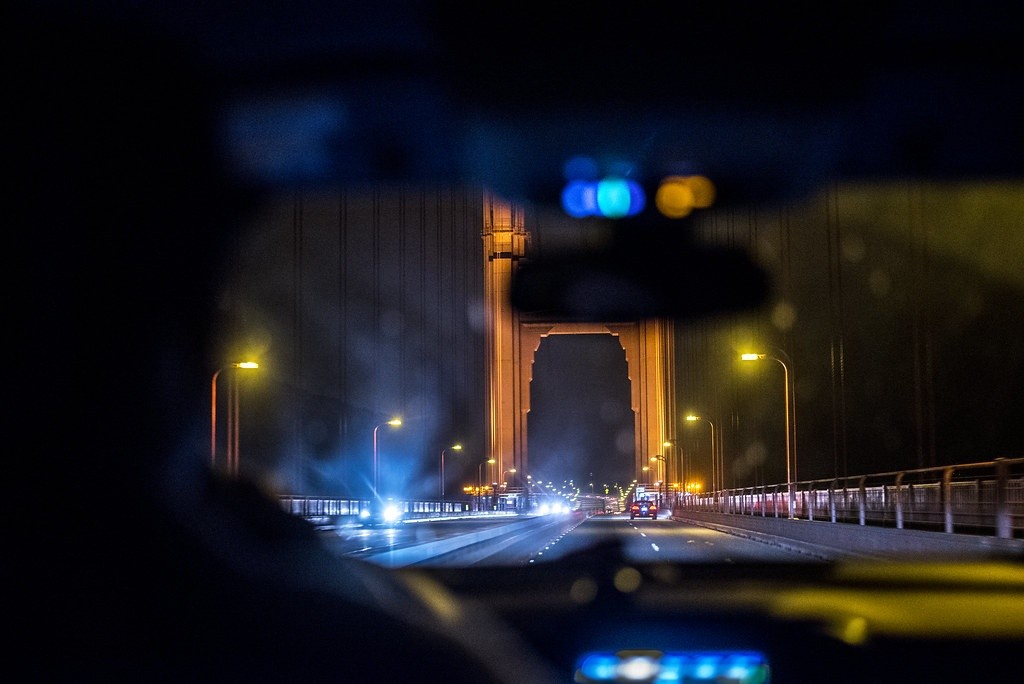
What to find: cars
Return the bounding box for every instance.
[629,500,658,520]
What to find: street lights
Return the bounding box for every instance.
[374,417,403,498]
[687,415,715,493]
[742,353,791,510]
[442,445,462,496]
[211,361,258,467]
[503,469,516,482]
[663,442,683,492]
[479,459,496,502]
